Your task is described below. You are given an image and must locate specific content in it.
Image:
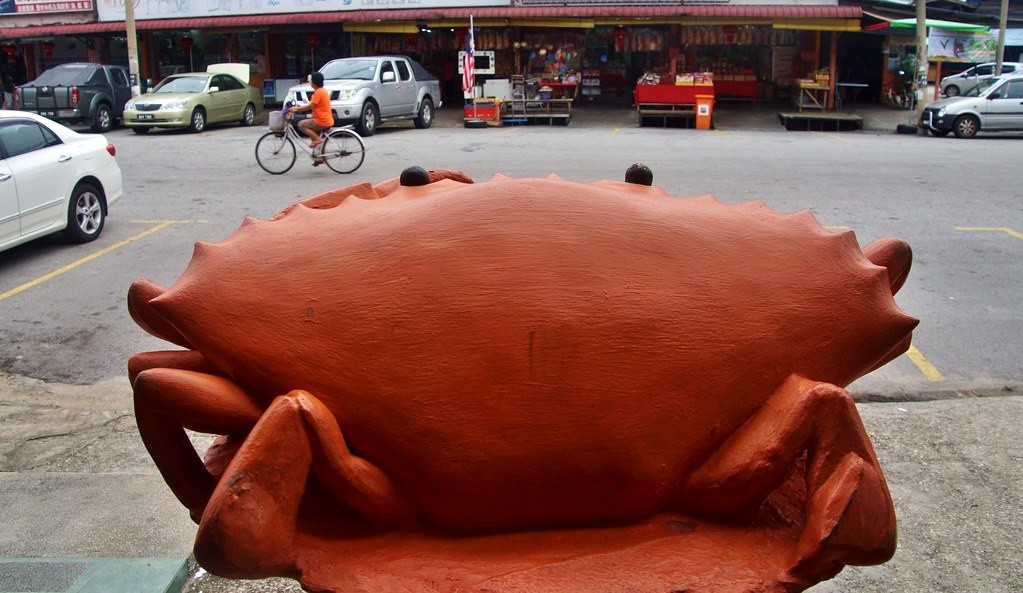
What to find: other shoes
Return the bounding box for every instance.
[310,139,323,148]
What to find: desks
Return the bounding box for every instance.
[635,85,715,128]
[792,86,830,112]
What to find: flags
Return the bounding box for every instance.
[462,15,476,93]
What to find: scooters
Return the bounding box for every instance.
[885,62,917,109]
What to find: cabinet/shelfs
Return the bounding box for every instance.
[500,98,576,118]
[581,56,604,107]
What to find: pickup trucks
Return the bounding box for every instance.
[14,63,152,132]
[282,56,442,137]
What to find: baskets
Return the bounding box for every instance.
[269,111,287,131]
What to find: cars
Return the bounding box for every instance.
[159,65,187,78]
[123,64,263,134]
[0,109,125,252]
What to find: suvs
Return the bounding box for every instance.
[940,62,1023,97]
[918,75,1023,139]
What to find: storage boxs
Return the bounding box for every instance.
[715,75,723,80]
[734,75,745,81]
[538,91,554,100]
[745,76,756,81]
[464,104,495,118]
[723,75,733,81]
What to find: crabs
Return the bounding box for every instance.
[126,166,920,592]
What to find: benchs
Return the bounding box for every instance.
[640,110,696,129]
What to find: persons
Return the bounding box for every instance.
[442,56,457,101]
[288,72,334,164]
[3,64,14,93]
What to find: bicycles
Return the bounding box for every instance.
[256,104,367,176]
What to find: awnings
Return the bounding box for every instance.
[889,18,990,34]
